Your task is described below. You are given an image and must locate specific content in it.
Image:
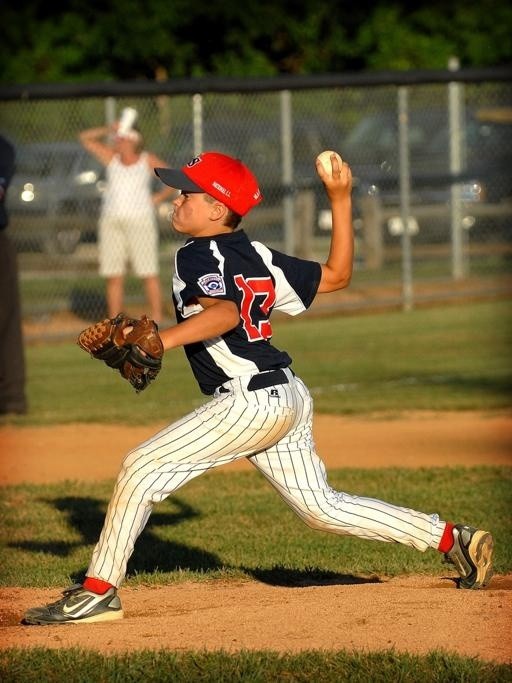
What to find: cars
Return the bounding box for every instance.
[3,102,511,257]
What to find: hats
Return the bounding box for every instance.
[154,149,262,216]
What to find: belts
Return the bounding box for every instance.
[214,364,295,394]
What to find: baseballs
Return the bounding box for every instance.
[318,151,342,175]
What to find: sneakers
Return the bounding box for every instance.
[445,523,494,589]
[20,584,125,625]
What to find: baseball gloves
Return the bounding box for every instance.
[78,314,163,391]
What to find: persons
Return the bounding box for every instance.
[21,149,496,626]
[78,120,175,328]
[0,134,28,419]
